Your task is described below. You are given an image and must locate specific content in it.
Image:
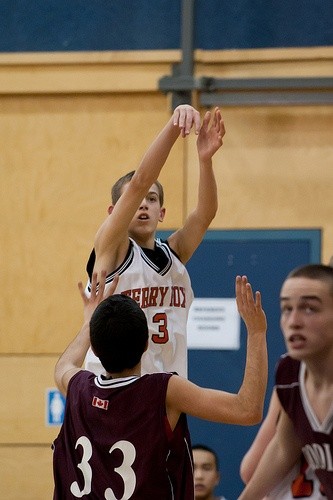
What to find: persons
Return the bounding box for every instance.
[51,268,268,500]
[84,105,226,382]
[237,256,333,500]
[191,444,227,500]
